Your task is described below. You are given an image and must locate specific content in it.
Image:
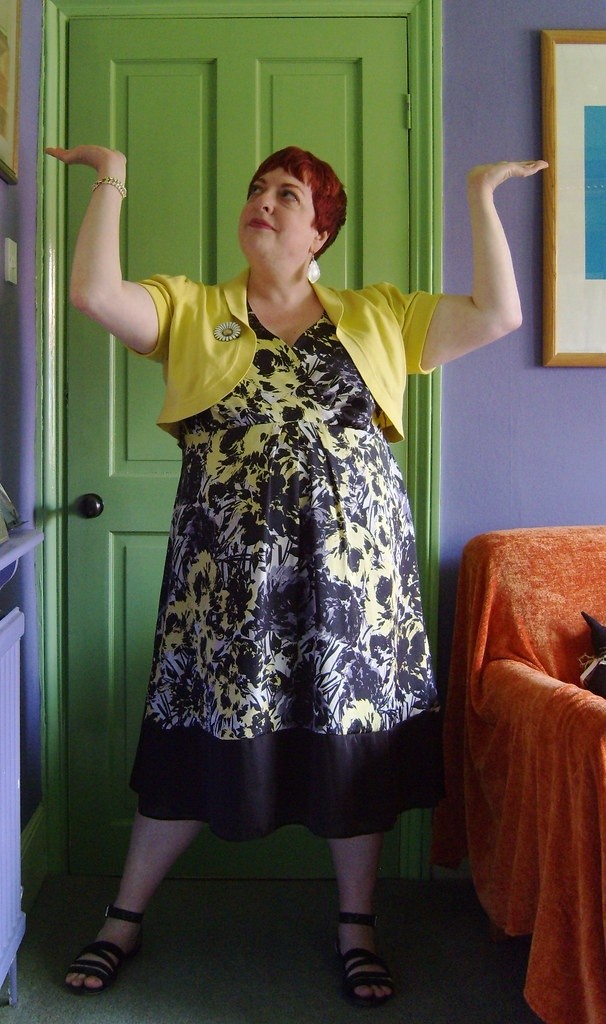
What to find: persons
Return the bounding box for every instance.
[44,144,549,1005]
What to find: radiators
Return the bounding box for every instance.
[0,607,27,991]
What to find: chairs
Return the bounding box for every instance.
[431,524,606,1024]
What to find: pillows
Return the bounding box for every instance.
[581,611,606,700]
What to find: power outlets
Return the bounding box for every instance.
[5,237,18,286]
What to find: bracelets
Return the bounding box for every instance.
[91,176,127,200]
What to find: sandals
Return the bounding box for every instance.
[333,912,396,1009]
[63,903,145,996]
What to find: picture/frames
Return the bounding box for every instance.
[0,0,22,186]
[541,29,606,368]
